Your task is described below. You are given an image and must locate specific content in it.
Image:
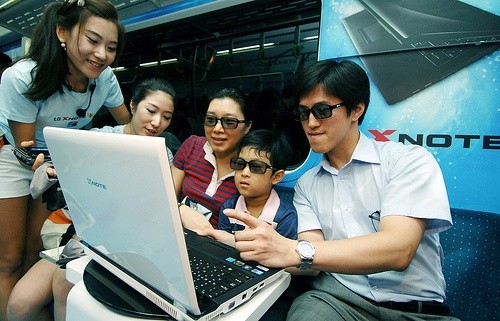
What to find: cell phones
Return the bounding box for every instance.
[14,146,51,165]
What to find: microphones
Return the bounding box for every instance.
[76,81,96,118]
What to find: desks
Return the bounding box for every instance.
[39,246,291,321]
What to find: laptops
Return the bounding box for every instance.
[43,126,285,321]
[342,0,500,105]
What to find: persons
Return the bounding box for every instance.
[8,81,181,318]
[2,2,129,319]
[171,89,252,233]
[223,60,452,321]
[216,131,299,239]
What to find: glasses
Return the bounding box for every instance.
[290,102,346,121]
[230,157,278,174]
[201,115,246,129]
[369,211,380,232]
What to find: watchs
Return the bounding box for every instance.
[295,238,318,273]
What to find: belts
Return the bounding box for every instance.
[378,300,450,316]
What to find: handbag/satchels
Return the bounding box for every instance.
[40,205,79,250]
